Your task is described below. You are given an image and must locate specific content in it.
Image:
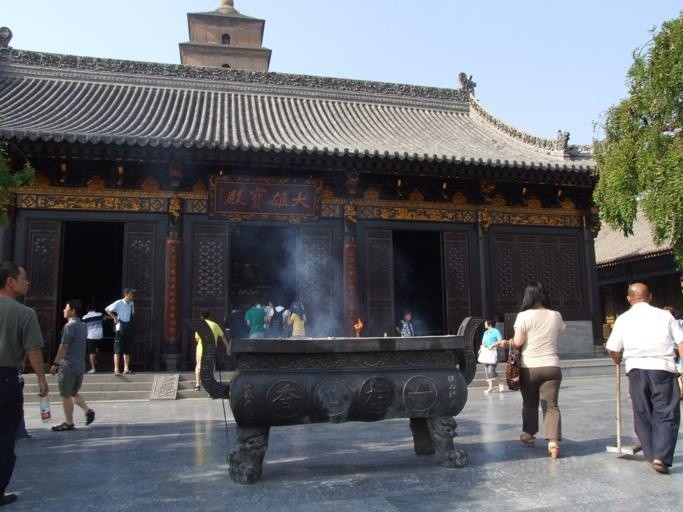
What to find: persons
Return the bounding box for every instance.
[624,305,682,405]
[497,279,569,460]
[477,314,506,396]
[49,286,138,432]
[193,302,309,399]
[605,282,682,474]
[393,310,416,337]
[0,261,49,506]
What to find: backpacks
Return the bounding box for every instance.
[269,307,287,333]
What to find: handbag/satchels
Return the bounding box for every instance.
[119,319,135,337]
[505,338,520,390]
[477,345,498,365]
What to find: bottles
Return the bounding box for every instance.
[39,395,51,424]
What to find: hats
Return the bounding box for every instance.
[124,287,137,293]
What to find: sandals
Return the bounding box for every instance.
[123,370,135,374]
[652,459,669,474]
[520,434,536,447]
[85,409,95,425]
[114,370,120,376]
[548,446,561,458]
[51,422,75,431]
